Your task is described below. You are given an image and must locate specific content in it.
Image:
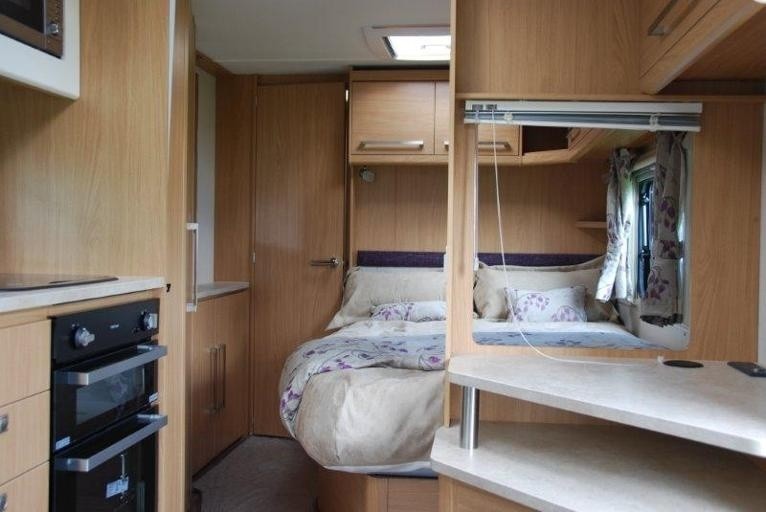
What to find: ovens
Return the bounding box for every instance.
[50,296,168,512]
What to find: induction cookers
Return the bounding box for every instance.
[0,268,121,291]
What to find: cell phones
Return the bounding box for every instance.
[726,361,766,377]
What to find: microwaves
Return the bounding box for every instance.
[0,0,65,60]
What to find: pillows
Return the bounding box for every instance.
[474,251,619,323]
[501,281,590,327]
[367,299,454,326]
[324,262,450,331]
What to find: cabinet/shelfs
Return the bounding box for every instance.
[565,119,594,152]
[346,77,522,160]
[427,348,766,512]
[190,288,252,479]
[0,317,56,511]
[519,122,572,157]
[638,0,765,95]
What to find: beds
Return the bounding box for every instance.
[272,247,691,511]
